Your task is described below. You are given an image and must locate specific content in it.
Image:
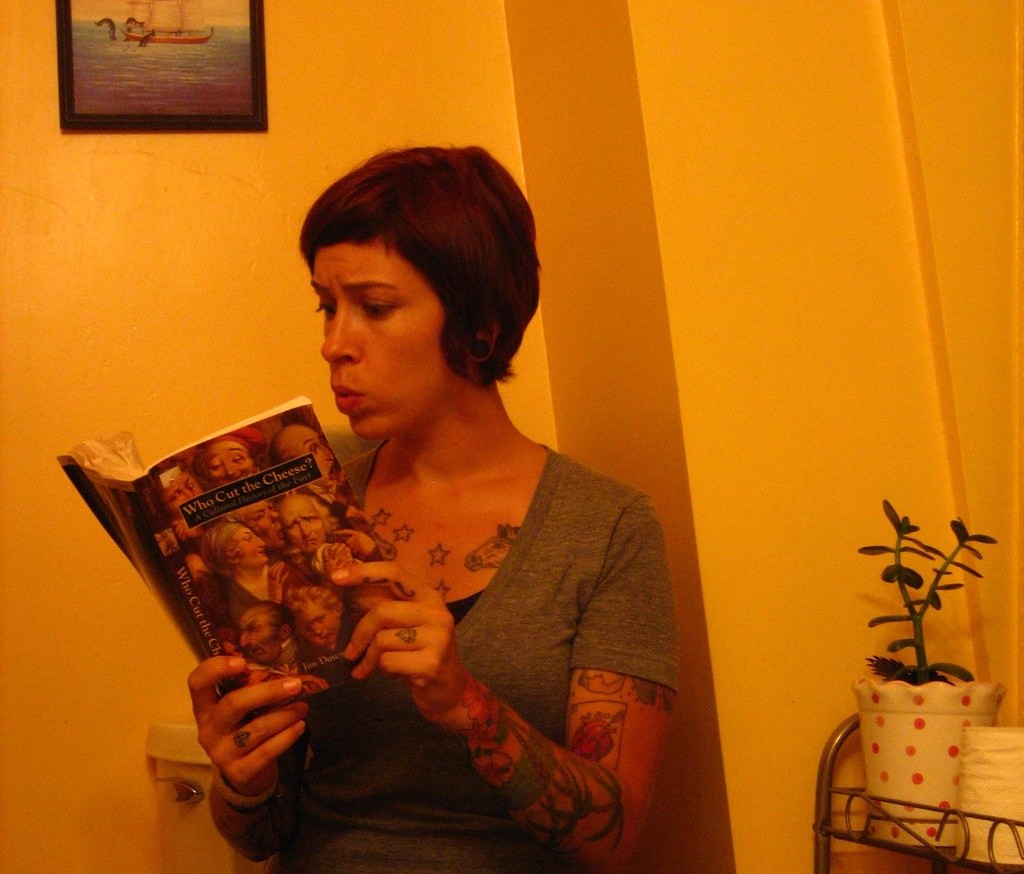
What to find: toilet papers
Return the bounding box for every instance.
[956,726,1024,865]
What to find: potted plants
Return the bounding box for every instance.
[850,499,1007,848]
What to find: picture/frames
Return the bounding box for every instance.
[55,0,268,132]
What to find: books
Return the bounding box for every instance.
[57,396,392,726]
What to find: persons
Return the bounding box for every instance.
[187,146,683,874]
